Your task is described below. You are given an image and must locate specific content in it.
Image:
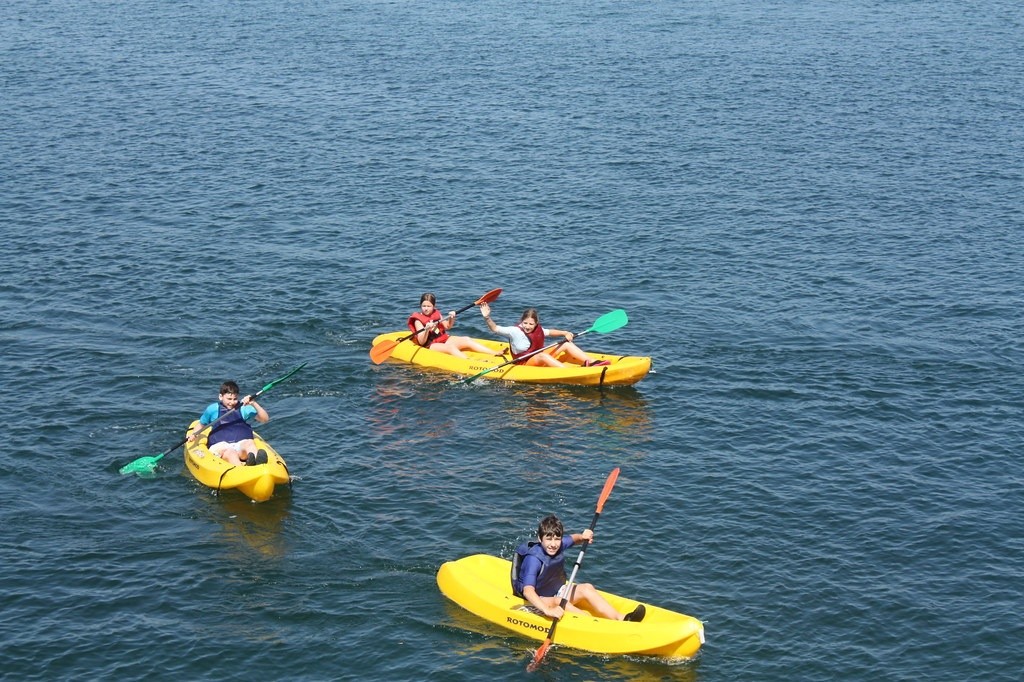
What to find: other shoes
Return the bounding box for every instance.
[581,359,610,367]
[244,449,268,466]
[624,604,645,622]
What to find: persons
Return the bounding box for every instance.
[478,300,612,367]
[509,516,648,623]
[187,382,271,466]
[408,293,509,361]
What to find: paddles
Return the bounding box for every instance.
[370,288,503,365]
[119,362,310,478]
[526,468,622,674]
[455,309,630,386]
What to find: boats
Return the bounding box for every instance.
[368,327,656,389]
[185,412,293,505]
[433,553,707,667]
